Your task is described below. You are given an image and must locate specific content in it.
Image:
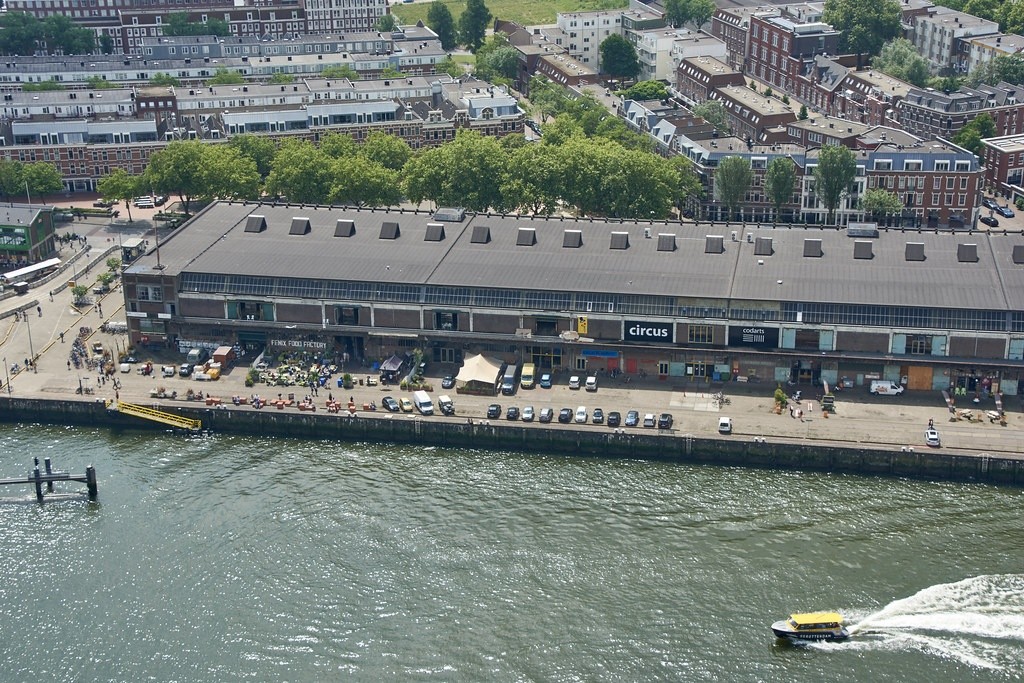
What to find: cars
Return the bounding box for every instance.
[981,217,999,226]
[575,406,587,423]
[179,364,193,376]
[608,412,621,425]
[488,404,501,418]
[382,396,399,411]
[643,413,655,426]
[559,408,572,421]
[925,429,941,447]
[592,408,604,423]
[399,398,412,412]
[540,372,554,388]
[569,375,581,389]
[522,406,534,420]
[164,365,175,376]
[442,373,455,387]
[718,416,732,432]
[981,196,1015,218]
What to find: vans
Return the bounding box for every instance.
[187,347,206,365]
[871,376,904,396]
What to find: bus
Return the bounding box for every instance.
[133,196,154,210]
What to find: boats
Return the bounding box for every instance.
[771,611,850,643]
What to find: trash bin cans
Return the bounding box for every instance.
[949,387,954,393]
[843,378,854,388]
[337,380,342,387]
[722,372,729,381]
[319,378,324,385]
[713,372,720,380]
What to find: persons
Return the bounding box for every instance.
[0,231,1009,429]
[42,199,162,221]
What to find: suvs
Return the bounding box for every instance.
[539,408,553,422]
[586,375,598,391]
[658,414,673,429]
[507,407,519,419]
[625,410,638,426]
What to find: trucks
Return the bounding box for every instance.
[521,363,537,389]
[206,344,236,380]
[501,365,518,395]
[413,390,435,414]
[439,395,455,414]
